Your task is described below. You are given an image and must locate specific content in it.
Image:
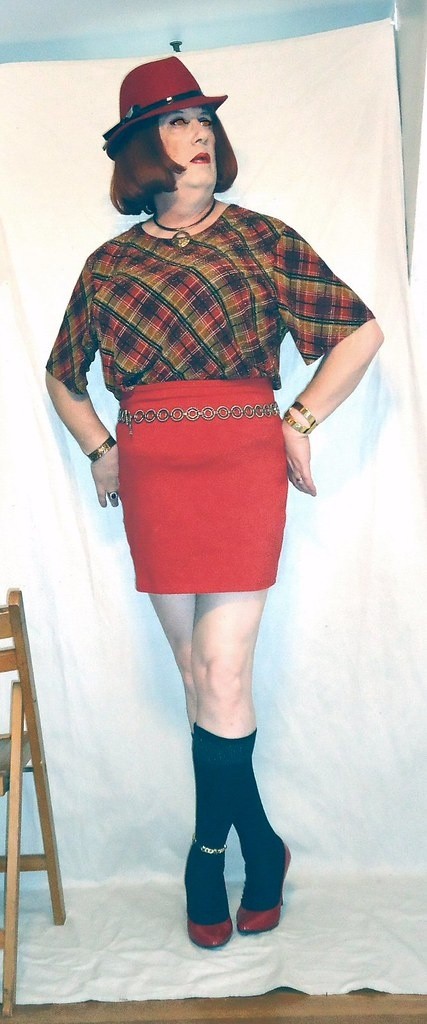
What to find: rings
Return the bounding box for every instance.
[297,478,303,481]
[108,492,118,500]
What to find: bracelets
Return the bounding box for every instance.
[87,435,117,462]
[284,402,317,435]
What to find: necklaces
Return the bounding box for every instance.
[152,198,216,248]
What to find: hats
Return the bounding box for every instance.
[103,56,228,161]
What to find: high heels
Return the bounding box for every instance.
[187,912,233,950]
[236,842,291,935]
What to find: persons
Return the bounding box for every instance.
[44,57,386,952]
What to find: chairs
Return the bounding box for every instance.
[0,591,67,1016]
